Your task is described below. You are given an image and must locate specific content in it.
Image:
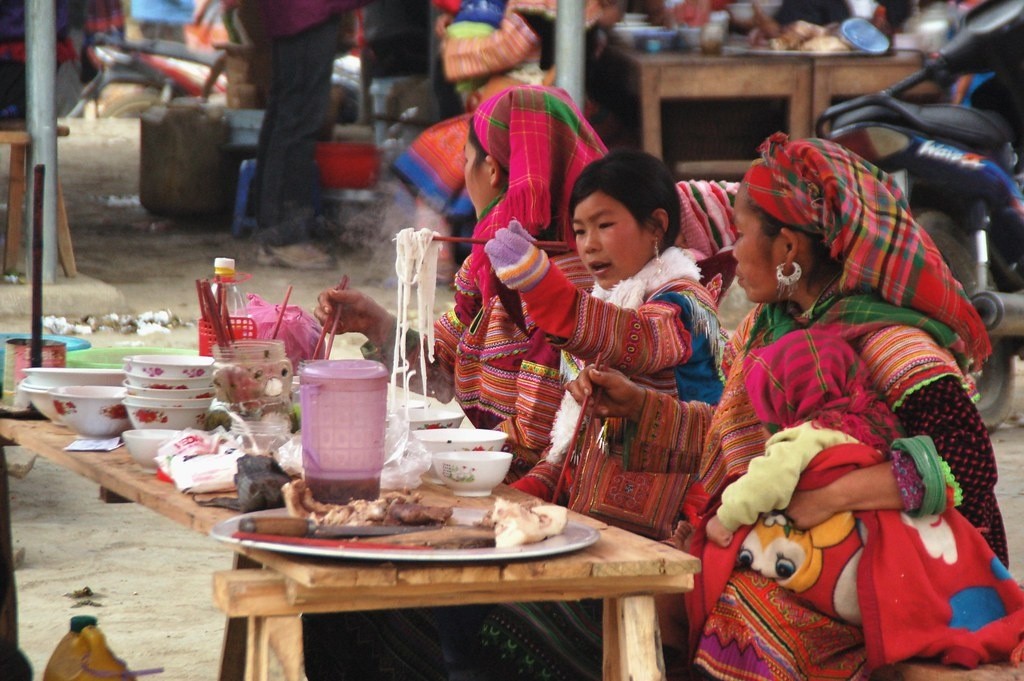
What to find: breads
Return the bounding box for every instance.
[492,496,568,549]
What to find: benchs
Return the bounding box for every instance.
[876,659,1024,681]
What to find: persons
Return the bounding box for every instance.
[239,0,365,267]
[393,0,1008,681]
[131,0,196,40]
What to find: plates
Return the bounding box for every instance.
[210,506,599,562]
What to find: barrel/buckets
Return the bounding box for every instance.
[141,105,230,217]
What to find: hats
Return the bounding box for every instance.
[675,177,741,257]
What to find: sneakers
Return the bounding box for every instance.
[256,238,338,270]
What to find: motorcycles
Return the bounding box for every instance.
[814,0,1024,433]
[66,33,394,119]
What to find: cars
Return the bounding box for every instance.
[187,0,365,55]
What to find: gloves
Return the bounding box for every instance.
[484,220,551,293]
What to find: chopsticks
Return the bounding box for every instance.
[310,276,349,358]
[551,352,611,507]
[190,278,293,389]
[434,235,569,252]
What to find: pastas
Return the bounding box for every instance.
[387,227,440,421]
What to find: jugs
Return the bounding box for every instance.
[296,359,388,507]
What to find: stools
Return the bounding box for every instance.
[0,120,77,276]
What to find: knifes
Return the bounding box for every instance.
[237,517,439,537]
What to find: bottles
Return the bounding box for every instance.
[210,257,248,319]
[230,419,294,457]
[211,340,293,413]
[43,615,129,680]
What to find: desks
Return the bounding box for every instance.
[815,49,950,158]
[0,406,700,681]
[604,34,814,183]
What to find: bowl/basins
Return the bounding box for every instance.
[19,353,214,475]
[729,4,773,19]
[315,142,385,190]
[322,191,392,241]
[609,12,703,50]
[381,398,514,499]
